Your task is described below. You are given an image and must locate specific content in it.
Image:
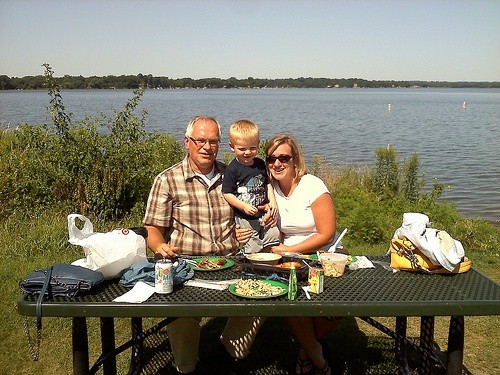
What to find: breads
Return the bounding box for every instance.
[280,262,302,268]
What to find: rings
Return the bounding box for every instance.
[239,232,241,236]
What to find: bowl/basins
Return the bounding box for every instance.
[246,253,282,266]
[320,253,347,277]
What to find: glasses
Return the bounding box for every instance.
[266,155,294,163]
[189,137,221,147]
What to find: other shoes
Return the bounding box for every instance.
[212,329,255,375]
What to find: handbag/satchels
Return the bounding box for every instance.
[390,238,472,275]
[18,263,104,300]
[67,214,147,279]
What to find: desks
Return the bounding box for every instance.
[17,255,500,375]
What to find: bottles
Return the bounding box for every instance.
[288,263,298,300]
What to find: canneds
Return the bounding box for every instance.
[154,257,173,294]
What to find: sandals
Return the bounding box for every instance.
[315,361,331,375]
[295,347,314,375]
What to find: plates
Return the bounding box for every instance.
[229,280,289,299]
[187,258,235,271]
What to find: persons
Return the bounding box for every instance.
[235,135,336,375]
[222,119,281,254]
[142,115,267,375]
